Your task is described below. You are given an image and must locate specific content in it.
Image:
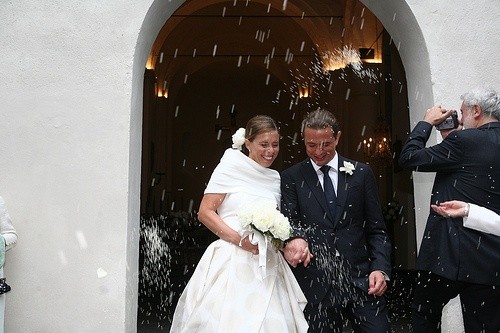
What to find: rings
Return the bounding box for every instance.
[443,214,448,217]
[299,259,303,263]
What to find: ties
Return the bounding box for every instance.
[320,165,337,221]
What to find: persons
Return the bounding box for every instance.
[169,115,314,333]
[430,199,500,236]
[280,108,394,333]
[0,194,18,333]
[398,84,500,333]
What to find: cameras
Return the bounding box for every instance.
[435,111,458,131]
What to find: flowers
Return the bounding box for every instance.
[231,127,246,151]
[339,160,356,183]
[242,202,294,255]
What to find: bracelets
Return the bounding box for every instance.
[464,203,470,217]
